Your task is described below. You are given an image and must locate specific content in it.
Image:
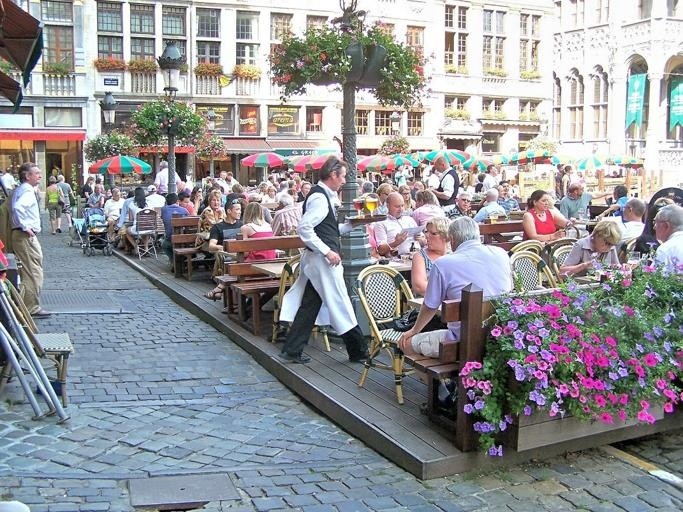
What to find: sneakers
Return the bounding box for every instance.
[32,309,51,318]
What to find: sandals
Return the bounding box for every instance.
[203,287,223,300]
[221,305,238,314]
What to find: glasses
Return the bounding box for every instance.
[381,193,388,195]
[401,193,408,195]
[422,230,440,235]
[460,198,470,202]
[229,199,242,204]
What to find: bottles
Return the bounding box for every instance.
[586,207,591,223]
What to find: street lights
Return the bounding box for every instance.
[155,40,186,194]
[99,91,118,186]
[202,107,220,177]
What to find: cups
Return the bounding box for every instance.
[490,214,497,224]
[627,251,641,263]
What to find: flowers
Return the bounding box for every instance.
[82,98,226,163]
[268,20,437,115]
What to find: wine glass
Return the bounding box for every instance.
[578,207,585,222]
[365,198,379,217]
[352,199,365,219]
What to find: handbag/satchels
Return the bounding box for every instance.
[393,308,449,332]
[58,195,64,205]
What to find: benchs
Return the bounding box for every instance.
[473,222,553,252]
[223,236,305,314]
[170,217,211,281]
[228,236,307,336]
[403,274,609,453]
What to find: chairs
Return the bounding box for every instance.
[134,209,159,260]
[271,250,332,353]
[0,277,76,409]
[356,265,425,405]
[510,237,580,291]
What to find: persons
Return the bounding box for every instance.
[162,153,683,418]
[1,155,51,317]
[45,174,78,235]
[81,161,182,256]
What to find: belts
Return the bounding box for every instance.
[12,227,22,231]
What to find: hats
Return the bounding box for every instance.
[147,185,156,191]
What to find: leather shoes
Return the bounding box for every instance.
[278,349,312,363]
[349,356,371,362]
